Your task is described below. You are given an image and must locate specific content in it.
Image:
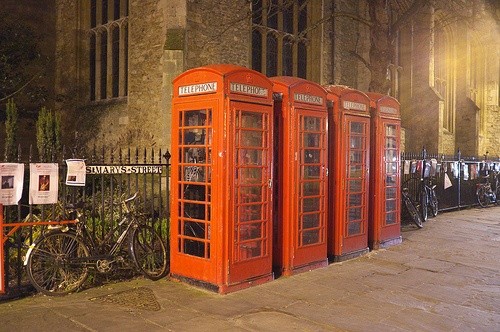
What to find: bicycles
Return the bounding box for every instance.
[23,190,168,297]
[400,177,424,228]
[475,170,500,208]
[411,176,438,222]
